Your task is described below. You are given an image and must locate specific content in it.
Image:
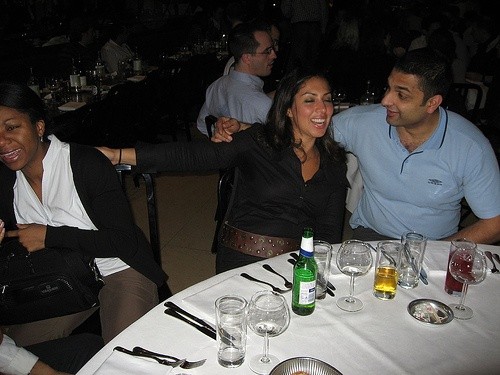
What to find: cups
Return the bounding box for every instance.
[181,37,229,54]
[332,89,375,115]
[398,231,427,289]
[373,241,402,301]
[444,237,477,296]
[214,294,248,369]
[312,239,332,300]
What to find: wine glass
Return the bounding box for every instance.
[448,247,488,320]
[336,239,373,312]
[45,76,61,105]
[246,290,290,375]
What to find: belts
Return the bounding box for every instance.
[217,220,298,258]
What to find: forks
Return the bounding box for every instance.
[241,264,292,294]
[485,250,500,274]
[113,345,207,369]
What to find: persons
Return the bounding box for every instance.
[197,22,277,137]
[211,48,500,244]
[95,71,352,274]
[0,83,169,345]
[0,333,104,375]
[67,0,500,115]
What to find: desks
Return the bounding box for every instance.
[32,58,182,143]
[180,37,231,97]
[72,240,500,375]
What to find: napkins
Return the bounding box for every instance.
[58,102,85,112]
[127,76,146,82]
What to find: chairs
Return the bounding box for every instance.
[70,163,174,335]
[270,42,294,77]
[448,83,484,131]
[209,167,236,254]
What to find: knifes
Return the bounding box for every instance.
[163,301,236,346]
[288,252,336,297]
[405,243,428,285]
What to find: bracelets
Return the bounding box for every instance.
[119,149,121,165]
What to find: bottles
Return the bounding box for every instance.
[291,228,317,316]
[68,45,142,98]
[27,63,40,97]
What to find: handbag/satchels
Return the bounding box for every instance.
[0,238,106,325]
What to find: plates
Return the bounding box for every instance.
[407,299,454,328]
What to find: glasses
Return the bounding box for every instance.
[240,47,273,58]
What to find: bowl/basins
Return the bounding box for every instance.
[269,357,343,375]
